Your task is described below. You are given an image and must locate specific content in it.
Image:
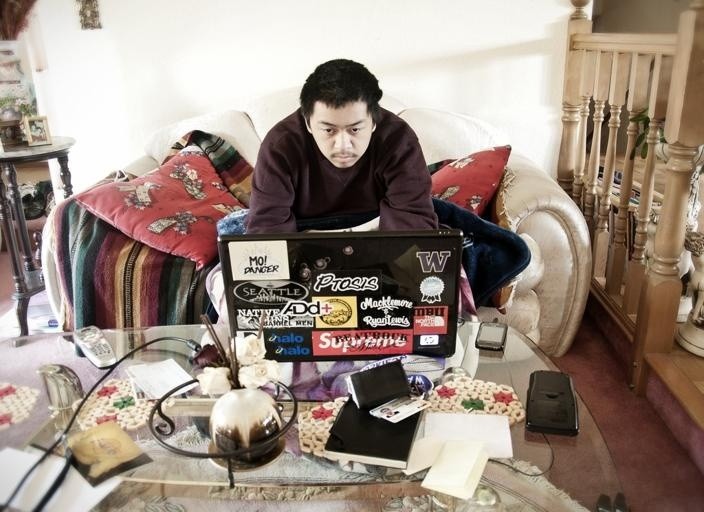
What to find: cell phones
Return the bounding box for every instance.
[525,369,580,436]
[474,321,508,351]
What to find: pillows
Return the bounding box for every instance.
[142,109,262,169]
[73,141,249,271]
[432,145,512,214]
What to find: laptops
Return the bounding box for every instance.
[215,228,465,364]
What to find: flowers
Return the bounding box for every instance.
[0,94,37,116]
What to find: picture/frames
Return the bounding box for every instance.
[24,115,52,146]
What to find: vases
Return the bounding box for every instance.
[0,109,22,122]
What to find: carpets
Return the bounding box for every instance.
[83,416,592,512]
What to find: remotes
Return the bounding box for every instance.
[72,323,118,370]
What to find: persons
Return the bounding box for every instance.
[37,127,43,133]
[380,406,399,418]
[30,124,43,141]
[246,57,440,234]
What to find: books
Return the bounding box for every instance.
[346,359,410,410]
[324,397,424,470]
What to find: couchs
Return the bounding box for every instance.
[40,94,593,358]
